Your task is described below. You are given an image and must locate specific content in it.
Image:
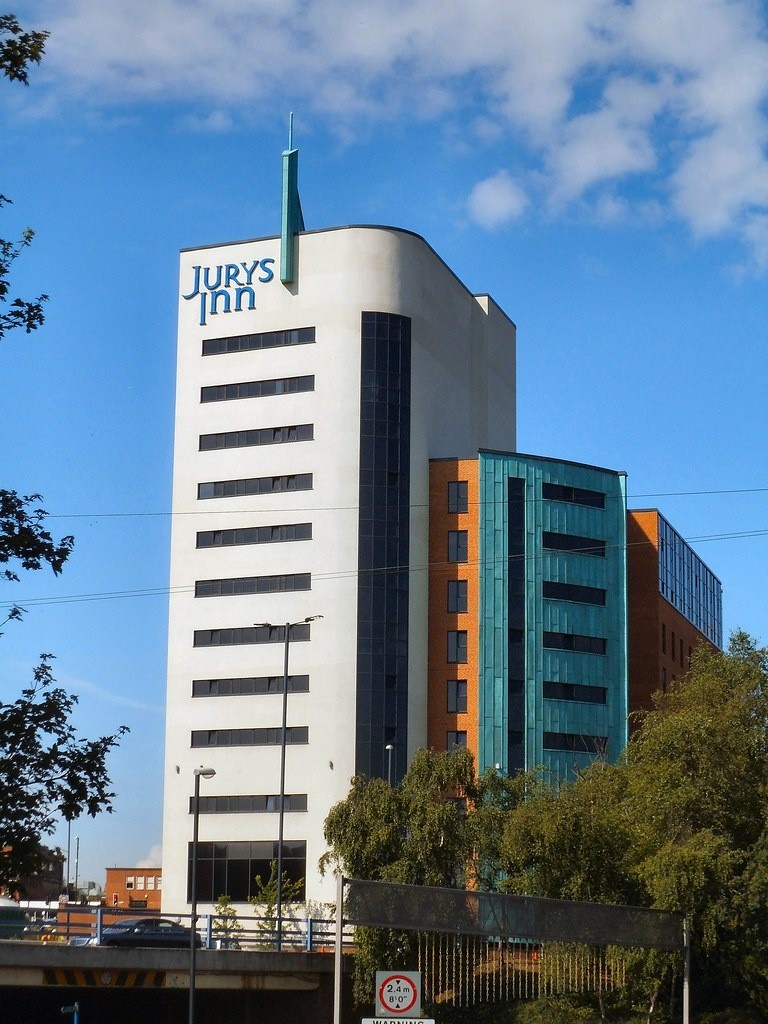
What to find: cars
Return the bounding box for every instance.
[66,918,205,951]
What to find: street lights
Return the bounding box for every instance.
[186,764,218,1024]
[384,743,397,787]
[66,818,72,889]
[74,836,81,896]
[253,614,326,952]
